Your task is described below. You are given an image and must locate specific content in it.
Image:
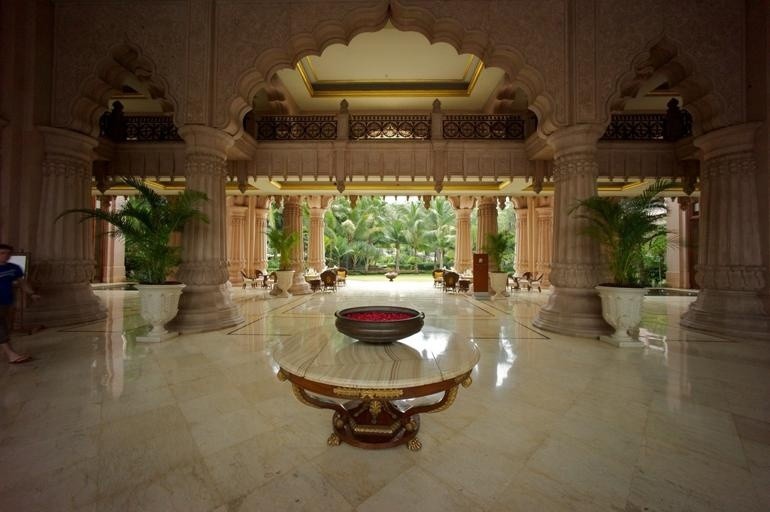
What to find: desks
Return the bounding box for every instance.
[275,322,482,452]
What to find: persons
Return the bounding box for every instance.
[0,244,42,363]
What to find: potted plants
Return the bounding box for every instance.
[564,176,702,348]
[478,229,517,300]
[52,174,213,343]
[259,227,301,299]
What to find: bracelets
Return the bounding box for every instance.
[29,292,35,297]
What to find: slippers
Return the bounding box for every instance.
[7,353,33,365]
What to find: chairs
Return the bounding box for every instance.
[240,268,347,293]
[433,269,544,294]
